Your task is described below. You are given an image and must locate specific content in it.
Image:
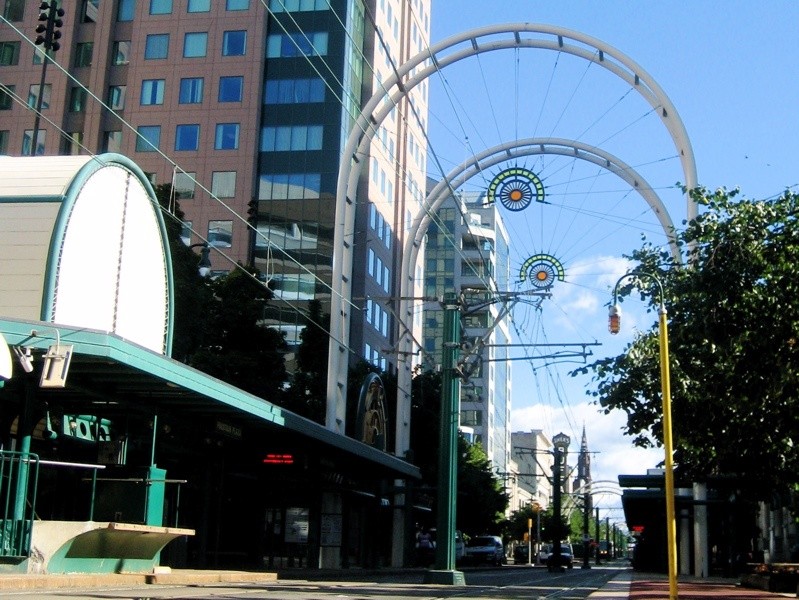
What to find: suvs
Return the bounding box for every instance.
[465,534,505,568]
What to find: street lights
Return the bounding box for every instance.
[28,0,66,156]
[608,271,678,600]
[536,511,546,565]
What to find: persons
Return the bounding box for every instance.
[416,524,432,568]
[495,544,503,567]
[552,539,562,573]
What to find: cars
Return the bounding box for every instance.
[512,539,613,570]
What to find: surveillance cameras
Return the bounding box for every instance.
[21,356,34,372]
